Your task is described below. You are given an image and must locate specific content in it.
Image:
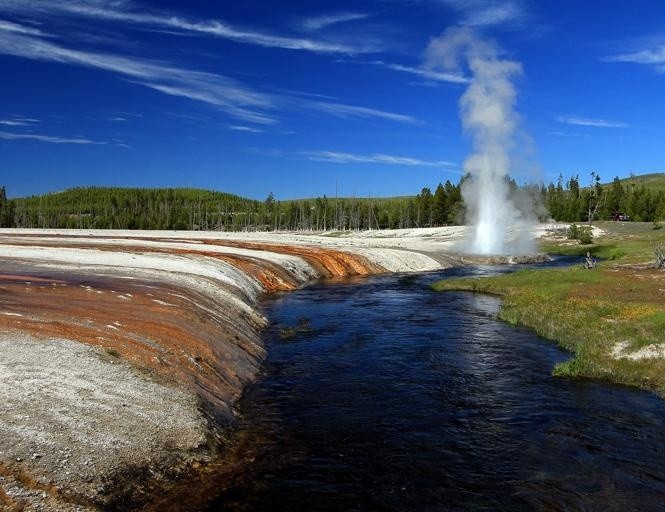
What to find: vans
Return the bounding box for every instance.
[608,212,630,222]
